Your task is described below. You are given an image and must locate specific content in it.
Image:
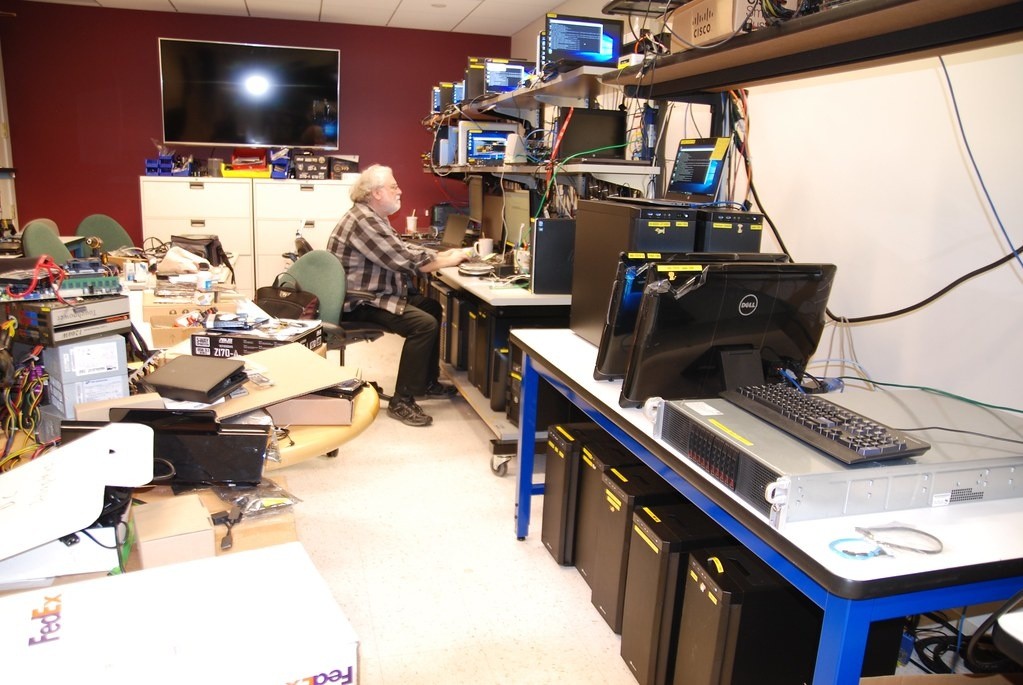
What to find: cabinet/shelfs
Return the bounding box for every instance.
[510,0,1023,685]
[406,65,662,477]
[139,175,256,304]
[252,178,354,288]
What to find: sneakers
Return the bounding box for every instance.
[388,395,433,426]
[414,381,457,400]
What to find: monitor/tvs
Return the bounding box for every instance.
[592,251,837,408]
[61,408,271,486]
[158,37,340,150]
[469,175,533,250]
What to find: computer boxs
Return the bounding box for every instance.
[569,199,697,346]
[529,218,575,295]
[425,276,907,685]
[694,206,764,253]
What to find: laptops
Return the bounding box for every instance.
[423,214,470,248]
[608,137,732,206]
[429,14,624,166]
[559,106,651,165]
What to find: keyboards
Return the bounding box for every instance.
[458,257,491,268]
[717,383,932,463]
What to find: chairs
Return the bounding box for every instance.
[253,237,394,402]
[76,214,134,256]
[0,218,73,274]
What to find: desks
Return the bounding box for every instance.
[58,236,85,258]
[0,430,296,598]
[264,382,380,470]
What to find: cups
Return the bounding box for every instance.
[406,216,418,235]
[513,250,530,274]
[474,238,494,260]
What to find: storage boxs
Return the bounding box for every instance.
[670,0,798,54]
[106,256,323,358]
[220,149,359,180]
[617,53,643,70]
[0,334,361,684]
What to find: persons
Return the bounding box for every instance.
[326,165,472,428]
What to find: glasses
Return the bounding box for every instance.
[380,184,398,191]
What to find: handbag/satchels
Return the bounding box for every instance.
[254,273,320,320]
